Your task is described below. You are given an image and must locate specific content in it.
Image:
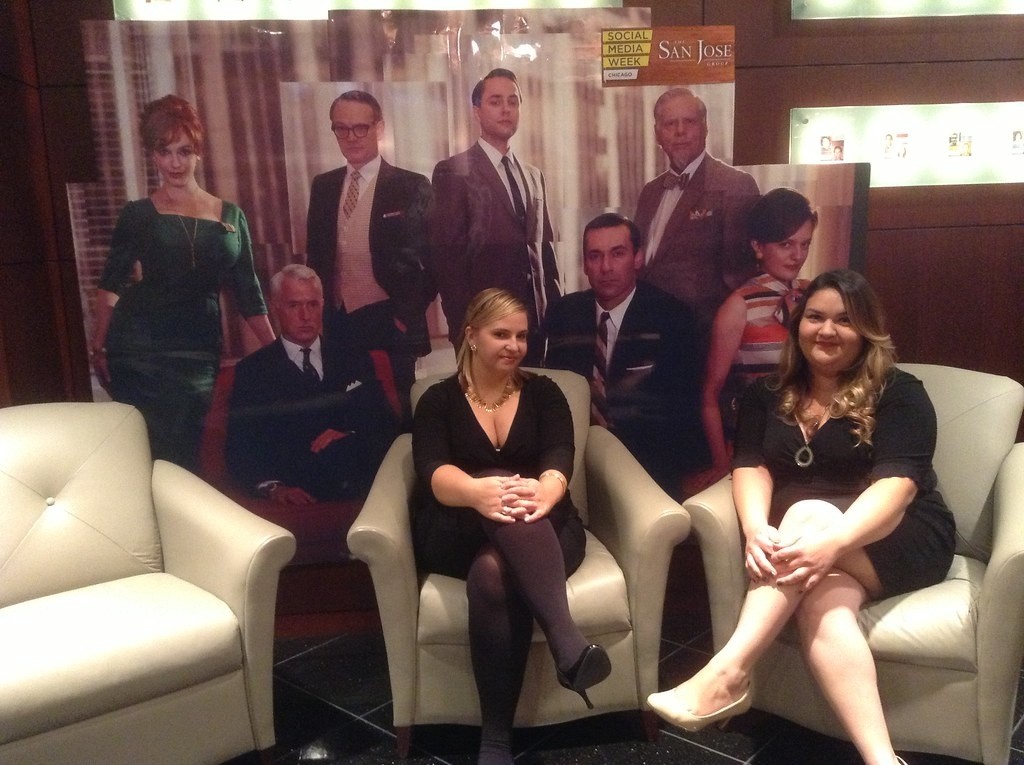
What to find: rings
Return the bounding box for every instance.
[502,506,512,515]
[499,496,506,507]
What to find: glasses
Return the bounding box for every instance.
[330,120,372,139]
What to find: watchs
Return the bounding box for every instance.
[261,482,281,498]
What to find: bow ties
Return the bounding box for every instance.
[663,173,690,190]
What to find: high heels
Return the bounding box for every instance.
[555,644,612,709]
[647,680,752,733]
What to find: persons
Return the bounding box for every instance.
[223,264,400,506]
[427,68,561,368]
[91,93,277,476]
[647,270,957,765]
[1013,131,1024,149]
[883,134,898,153]
[304,91,440,458]
[545,214,702,495]
[821,136,843,162]
[632,88,759,462]
[948,133,972,157]
[409,287,610,765]
[690,187,819,490]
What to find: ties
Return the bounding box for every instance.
[343,170,362,219]
[592,312,610,428]
[501,156,526,222]
[299,347,320,381]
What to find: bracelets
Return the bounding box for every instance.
[539,472,566,493]
[91,343,107,360]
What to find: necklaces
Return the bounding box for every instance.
[163,181,200,269]
[795,392,840,466]
[465,379,515,413]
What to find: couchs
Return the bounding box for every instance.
[0,349,1024,765]
